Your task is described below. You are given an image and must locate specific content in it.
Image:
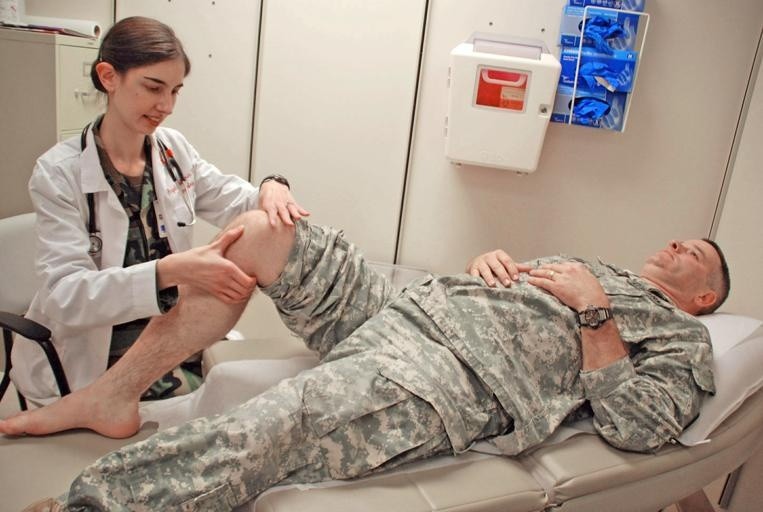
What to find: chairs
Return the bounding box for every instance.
[0,214,242,409]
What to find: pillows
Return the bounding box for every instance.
[673,314,762,448]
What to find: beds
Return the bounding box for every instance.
[1,314,763,512]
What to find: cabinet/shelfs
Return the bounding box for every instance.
[1,28,110,217]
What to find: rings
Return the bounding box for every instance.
[287,201,294,205]
[549,270,555,279]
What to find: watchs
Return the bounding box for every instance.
[578,303,613,329]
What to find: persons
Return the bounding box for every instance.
[7,15,310,408]
[0,208,731,512]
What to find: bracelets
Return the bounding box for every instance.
[260,174,291,190]
